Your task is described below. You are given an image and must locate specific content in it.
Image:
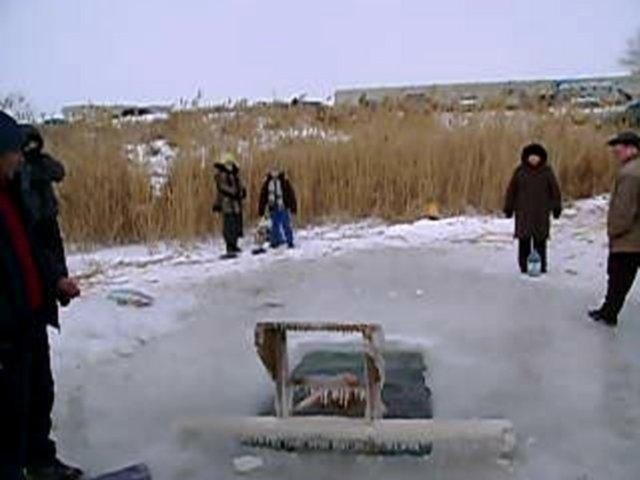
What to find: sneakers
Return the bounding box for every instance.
[0,453,84,480]
[588,309,618,327]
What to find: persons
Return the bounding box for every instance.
[6,122,80,341]
[586,131,639,327]
[501,143,562,275]
[1,108,88,479]
[256,162,297,250]
[211,154,248,258]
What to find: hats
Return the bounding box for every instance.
[606,130,640,148]
[0,111,25,152]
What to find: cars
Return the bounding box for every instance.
[456,94,484,112]
[110,104,170,127]
[542,82,639,127]
